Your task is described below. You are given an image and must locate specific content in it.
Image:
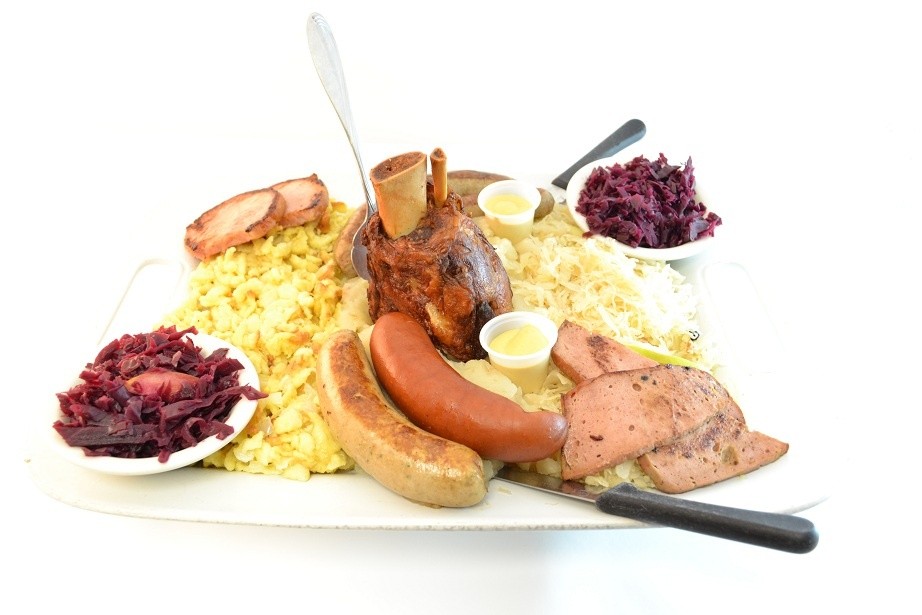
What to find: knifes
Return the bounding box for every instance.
[493,464,819,557]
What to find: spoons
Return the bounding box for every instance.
[304,11,388,281]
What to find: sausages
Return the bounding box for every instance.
[319,312,565,510]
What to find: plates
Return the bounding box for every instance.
[37,207,822,536]
[566,153,733,259]
